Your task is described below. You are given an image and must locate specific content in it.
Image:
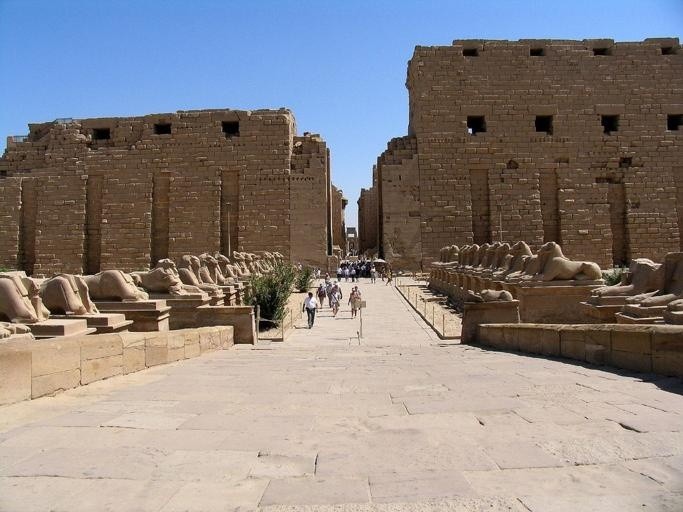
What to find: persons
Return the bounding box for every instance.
[297,259,393,329]
